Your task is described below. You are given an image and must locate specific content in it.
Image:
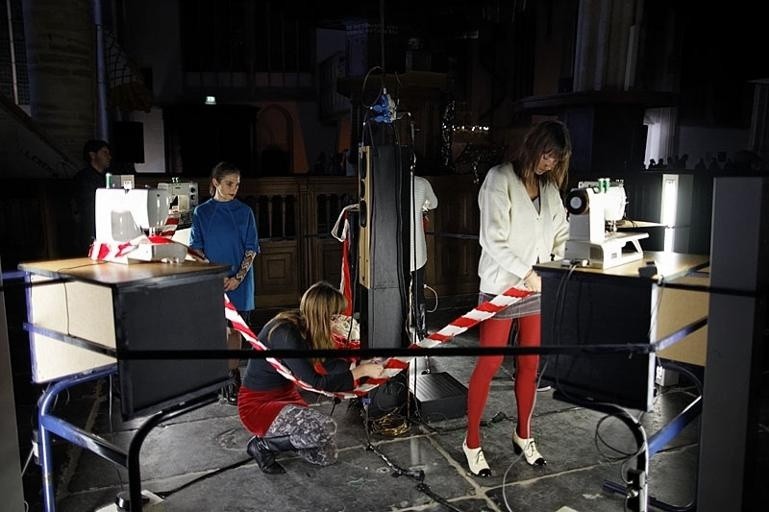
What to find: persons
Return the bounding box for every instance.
[461,118,571,478]
[188,161,263,405]
[236,281,387,473]
[70,140,112,256]
[408,157,440,336]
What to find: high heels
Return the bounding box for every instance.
[222,369,241,405]
[512,428,545,467]
[461,437,490,477]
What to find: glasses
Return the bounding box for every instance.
[329,315,340,321]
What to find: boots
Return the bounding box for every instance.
[247,433,299,475]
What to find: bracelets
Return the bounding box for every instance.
[524,270,534,283]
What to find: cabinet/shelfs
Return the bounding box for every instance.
[197,176,479,309]
